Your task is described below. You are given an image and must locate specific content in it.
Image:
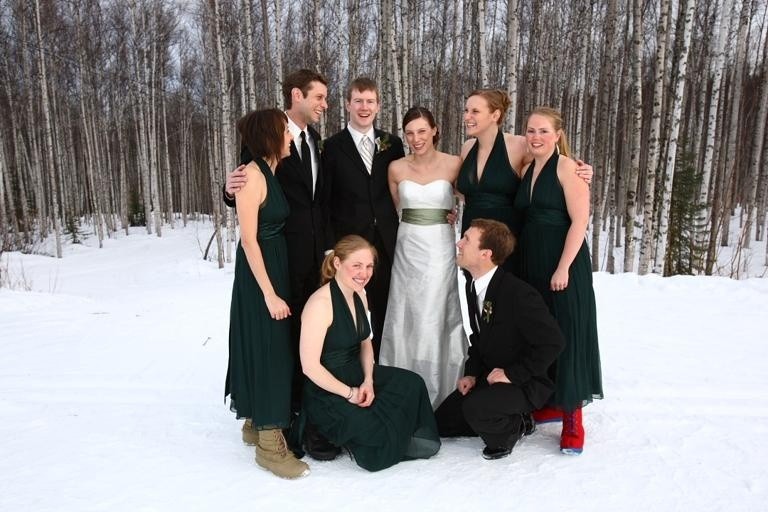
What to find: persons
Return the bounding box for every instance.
[223,68,329,357]
[321,78,457,365]
[223,108,309,479]
[518,107,604,456]
[432,218,568,459]
[454,88,594,284]
[378,105,464,412]
[285,234,442,472]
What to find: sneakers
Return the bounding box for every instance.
[302,419,337,460]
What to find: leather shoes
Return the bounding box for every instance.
[483,411,535,458]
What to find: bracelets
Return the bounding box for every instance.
[347,387,353,401]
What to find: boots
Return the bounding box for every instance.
[242,418,312,479]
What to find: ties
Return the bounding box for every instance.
[362,136,373,175]
[300,130,313,203]
[468,281,480,338]
